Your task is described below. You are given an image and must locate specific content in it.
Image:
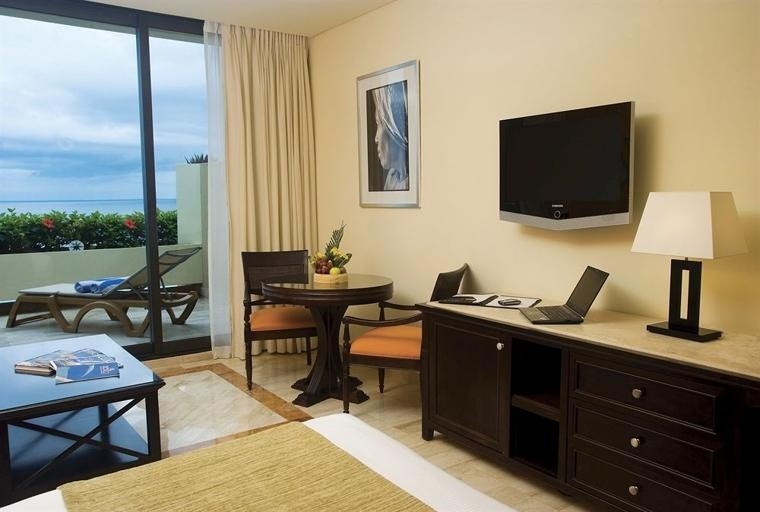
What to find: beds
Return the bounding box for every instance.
[1,413,520,511]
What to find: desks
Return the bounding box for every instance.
[262,274,394,407]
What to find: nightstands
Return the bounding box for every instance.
[416,297,759,512]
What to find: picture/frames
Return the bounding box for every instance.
[355,57,420,209]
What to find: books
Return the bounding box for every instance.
[14,370,56,377]
[49,348,123,385]
[15,349,64,372]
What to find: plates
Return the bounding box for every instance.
[313,272,348,284]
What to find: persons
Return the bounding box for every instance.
[372,82,408,191]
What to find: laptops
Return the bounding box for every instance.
[518,262,611,326]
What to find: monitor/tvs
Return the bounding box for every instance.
[499,100,637,232]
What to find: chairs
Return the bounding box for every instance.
[343,264,469,414]
[242,251,317,391]
[7,245,202,336]
